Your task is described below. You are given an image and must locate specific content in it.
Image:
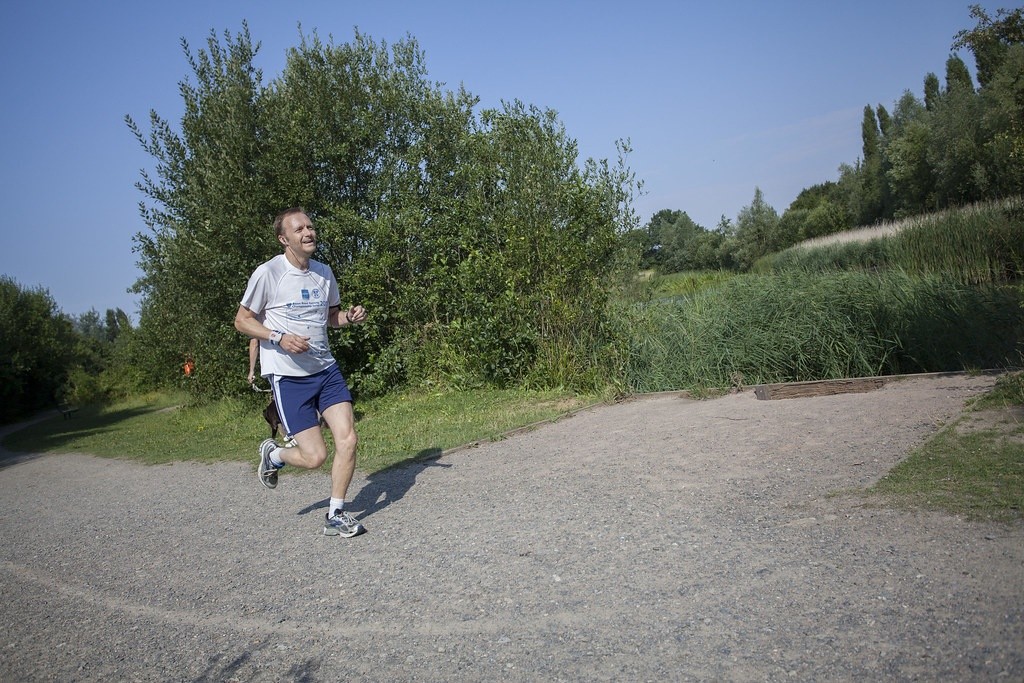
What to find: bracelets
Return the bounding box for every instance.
[346,313,354,324]
[270,330,285,345]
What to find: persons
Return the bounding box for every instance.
[235,209,367,538]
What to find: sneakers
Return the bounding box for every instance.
[325,508,365,538]
[258,438,284,489]
